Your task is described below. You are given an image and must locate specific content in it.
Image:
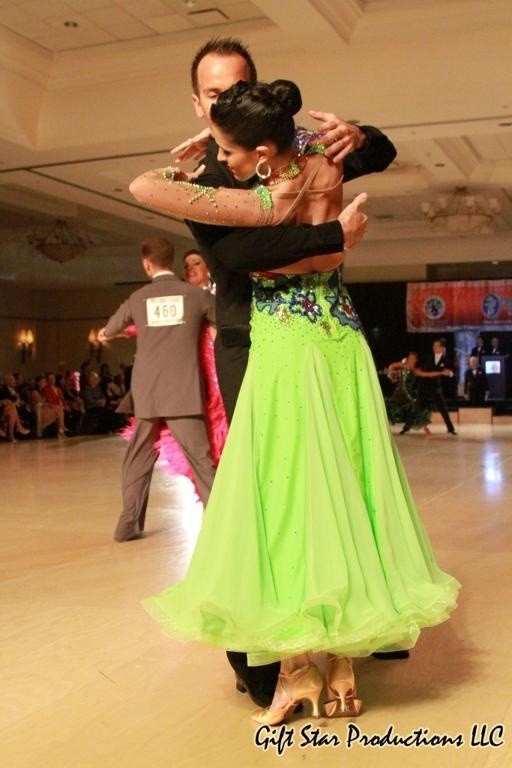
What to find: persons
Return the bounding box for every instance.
[98,235,219,540]
[99,249,229,503]
[126,76,466,725]
[486,338,503,354]
[2,358,133,445]
[383,350,443,435]
[398,337,457,436]
[462,355,487,393]
[185,43,412,714]
[470,337,490,367]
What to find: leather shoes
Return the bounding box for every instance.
[16,427,30,435]
[372,643,409,660]
[234,666,304,714]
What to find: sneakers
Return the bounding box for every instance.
[400,426,457,438]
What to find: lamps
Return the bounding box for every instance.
[18,329,34,364]
[88,328,104,363]
[420,185,501,239]
[27,219,94,264]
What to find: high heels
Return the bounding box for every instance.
[251,661,325,726]
[323,653,364,718]
